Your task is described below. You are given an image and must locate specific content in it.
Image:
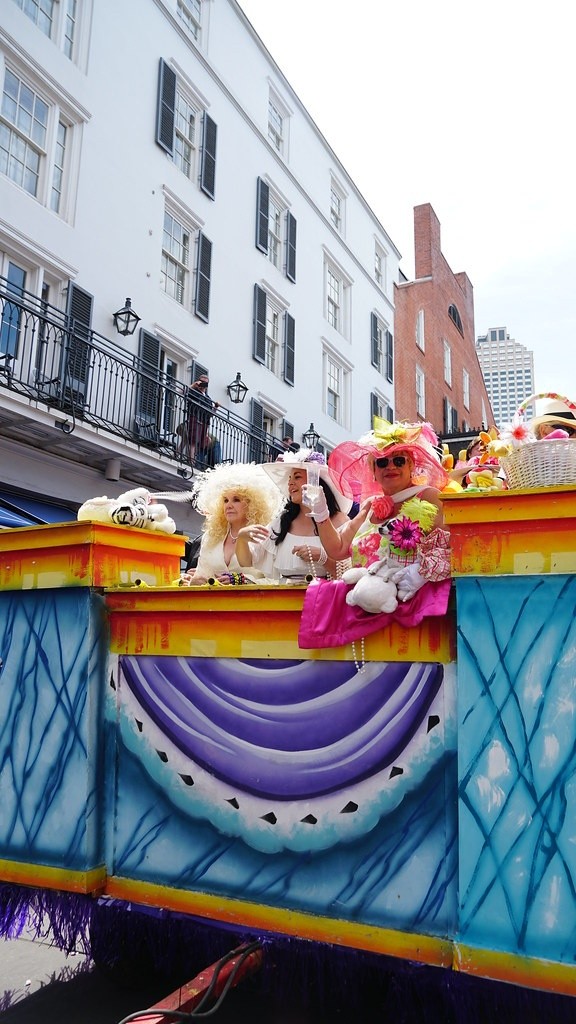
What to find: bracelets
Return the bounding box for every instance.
[316,548,328,565]
[222,572,245,586]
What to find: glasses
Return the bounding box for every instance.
[375,457,408,468]
[549,424,575,436]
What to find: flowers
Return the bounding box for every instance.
[499,418,536,452]
[307,452,326,465]
[388,517,425,554]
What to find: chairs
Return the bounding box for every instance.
[134,415,177,458]
[0,353,14,388]
[33,369,87,419]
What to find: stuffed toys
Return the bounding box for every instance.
[342,559,398,615]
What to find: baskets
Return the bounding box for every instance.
[499,392,576,487]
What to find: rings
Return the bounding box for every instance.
[250,532,253,538]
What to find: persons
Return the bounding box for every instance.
[180,449,443,585]
[178,375,221,471]
[455,435,505,490]
[283,437,300,453]
[498,401,576,489]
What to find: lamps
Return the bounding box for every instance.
[302,423,321,449]
[227,372,250,404]
[111,298,142,337]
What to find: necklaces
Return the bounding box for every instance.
[326,557,365,675]
[229,529,238,542]
[304,545,318,582]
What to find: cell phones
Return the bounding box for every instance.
[199,382,208,387]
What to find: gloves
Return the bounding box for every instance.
[300,483,330,522]
[397,563,427,603]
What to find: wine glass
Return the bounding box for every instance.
[305,464,321,517]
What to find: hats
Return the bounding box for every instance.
[329,416,449,498]
[262,449,353,515]
[466,436,481,461]
[528,401,576,431]
[449,457,502,484]
